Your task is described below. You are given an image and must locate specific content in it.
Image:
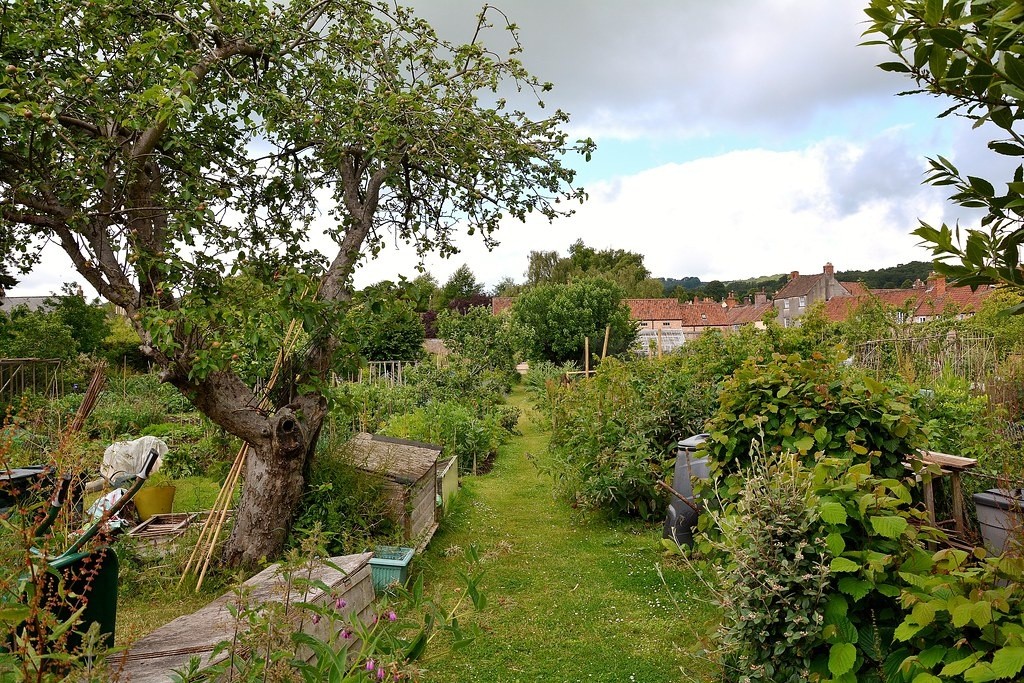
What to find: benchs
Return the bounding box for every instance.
[902,449,978,552]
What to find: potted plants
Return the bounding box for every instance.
[360,444,423,597]
